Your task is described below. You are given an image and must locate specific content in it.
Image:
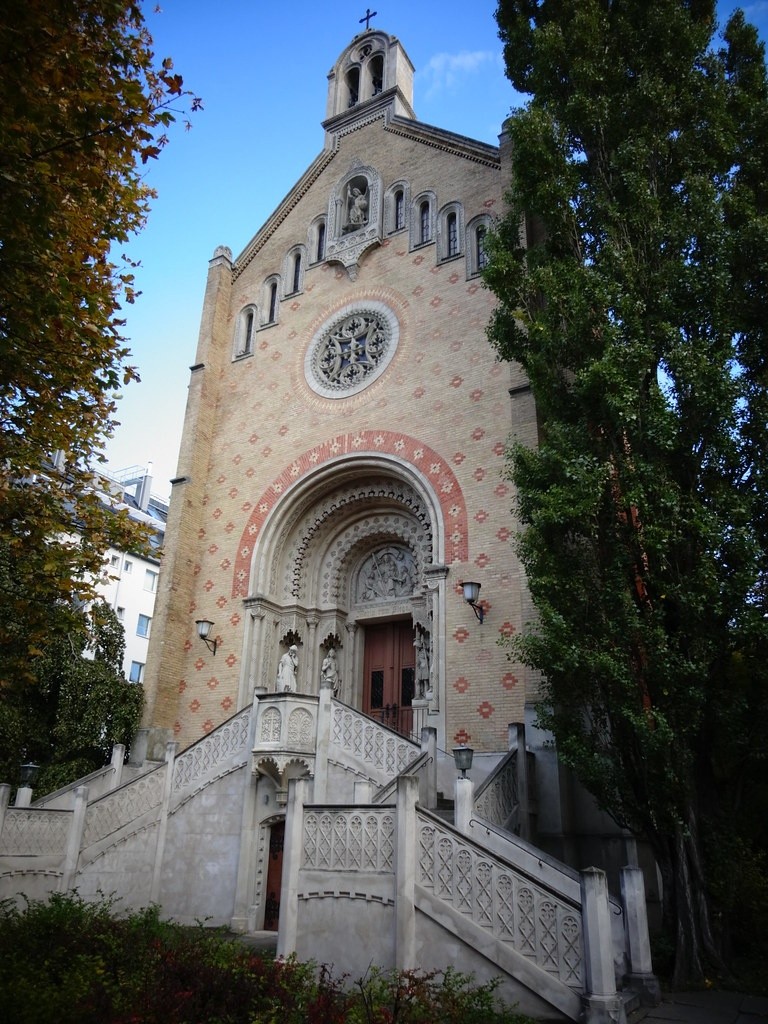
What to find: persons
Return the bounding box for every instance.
[358,555,412,601]
[277,644,299,693]
[321,648,339,697]
[348,185,368,223]
[413,640,429,699]
